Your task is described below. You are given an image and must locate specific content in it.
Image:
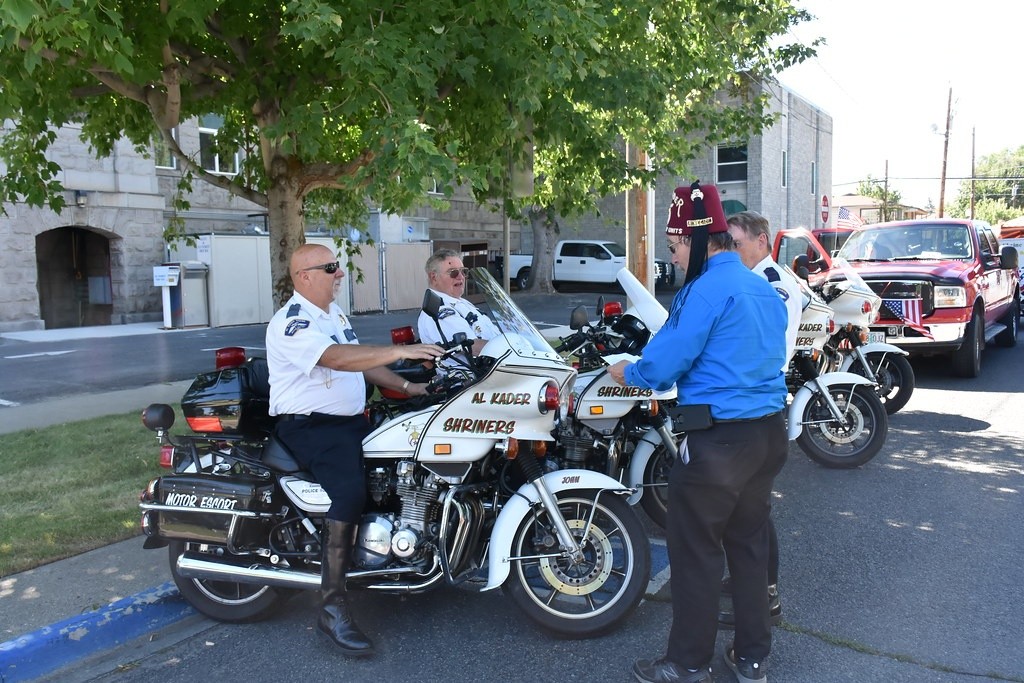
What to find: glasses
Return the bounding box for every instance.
[295,261,339,274]
[436,268,469,278]
[667,235,691,253]
[733,232,769,248]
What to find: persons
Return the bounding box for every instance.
[716,210,802,630]
[418,250,501,365]
[607,182,789,683]
[264,244,446,656]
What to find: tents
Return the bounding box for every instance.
[991,216,1024,314]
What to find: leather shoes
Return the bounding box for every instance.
[721,574,732,591]
[717,591,783,627]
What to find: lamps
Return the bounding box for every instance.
[76,190,88,208]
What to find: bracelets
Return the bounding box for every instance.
[402,381,409,394]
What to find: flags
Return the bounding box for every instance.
[883,300,934,341]
[837,207,865,230]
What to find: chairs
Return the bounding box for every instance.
[869,232,907,259]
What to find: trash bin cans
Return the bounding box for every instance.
[161,260,209,328]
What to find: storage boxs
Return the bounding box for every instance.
[376,338,437,404]
[157,474,274,554]
[180,356,273,436]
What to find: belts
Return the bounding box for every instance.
[714,410,780,423]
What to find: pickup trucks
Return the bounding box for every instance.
[494,240,675,295]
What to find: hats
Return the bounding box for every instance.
[666,180,728,330]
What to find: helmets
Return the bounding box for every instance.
[610,305,655,346]
[474,333,534,371]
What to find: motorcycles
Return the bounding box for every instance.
[139,265,653,637]
[360,254,915,532]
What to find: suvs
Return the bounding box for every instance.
[771,218,1021,379]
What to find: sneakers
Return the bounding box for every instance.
[632,655,716,683]
[723,640,767,683]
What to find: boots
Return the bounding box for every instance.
[315,518,376,655]
